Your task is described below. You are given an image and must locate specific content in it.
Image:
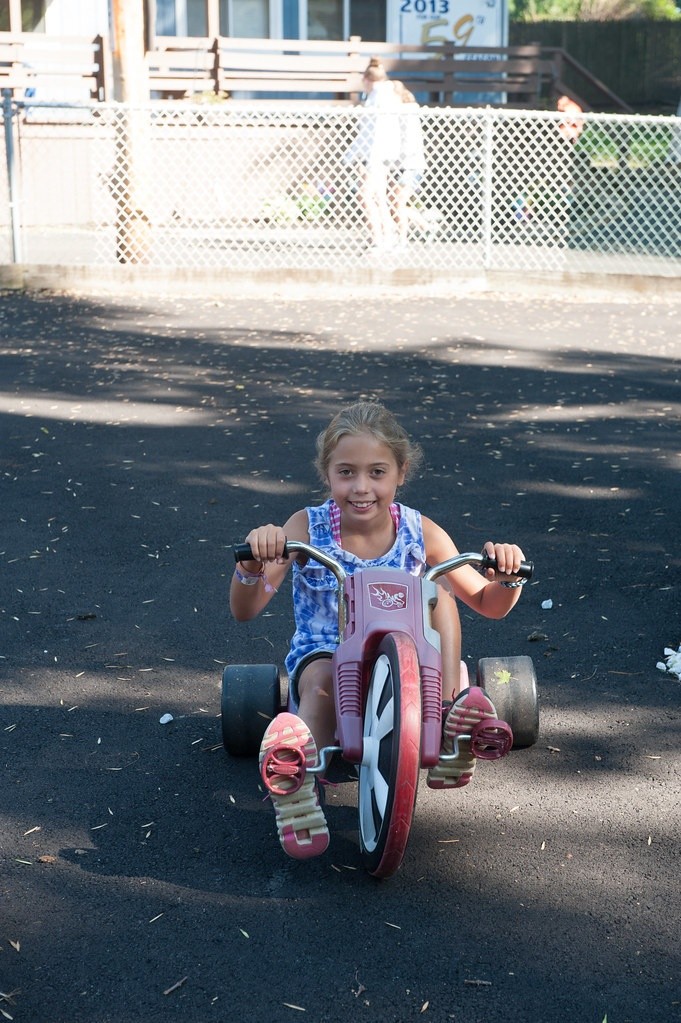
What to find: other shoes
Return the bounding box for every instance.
[425,686,498,790]
[258,712,331,860]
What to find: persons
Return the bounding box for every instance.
[229,401,528,860]
[347,56,426,253]
[550,92,583,145]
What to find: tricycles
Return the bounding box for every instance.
[221,536,545,870]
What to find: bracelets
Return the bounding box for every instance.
[497,577,528,589]
[235,561,278,593]
